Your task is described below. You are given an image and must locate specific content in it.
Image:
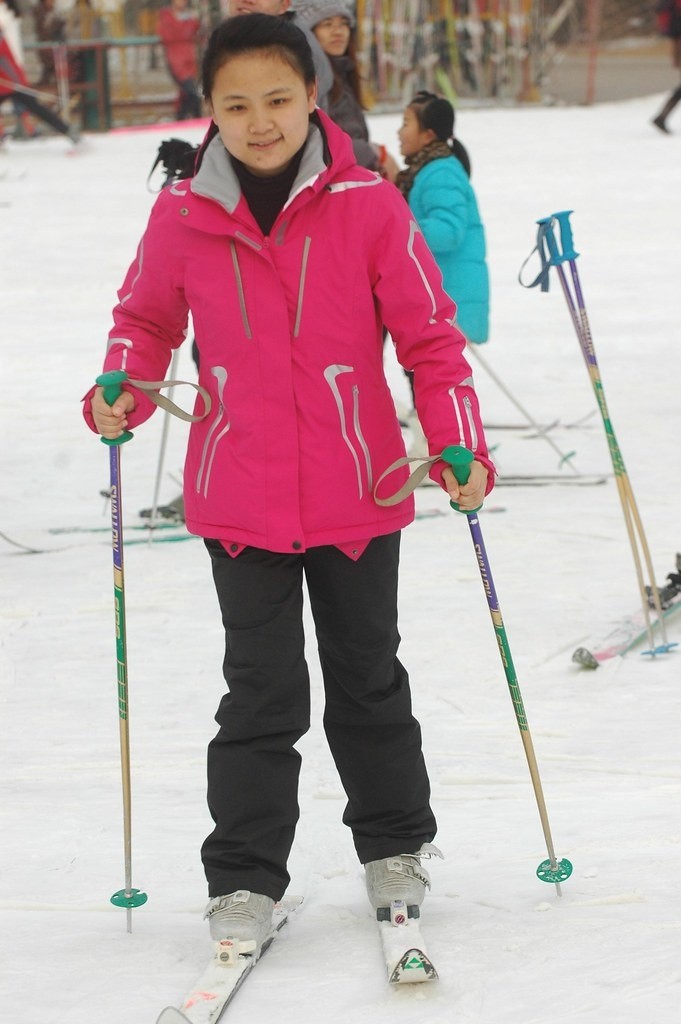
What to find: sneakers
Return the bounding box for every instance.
[200,887,276,951]
[362,842,445,922]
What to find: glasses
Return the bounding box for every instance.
[318,21,353,31]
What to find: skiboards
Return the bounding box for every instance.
[155,896,288,1024]
[417,466,607,488]
[374,902,437,988]
[48,513,200,548]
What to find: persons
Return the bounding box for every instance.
[387,89,491,470]
[158,0,205,122]
[0,1,84,150]
[294,0,400,185]
[80,10,498,954]
[137,0,333,521]
[653,0,681,133]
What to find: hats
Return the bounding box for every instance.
[296,0,357,28]
